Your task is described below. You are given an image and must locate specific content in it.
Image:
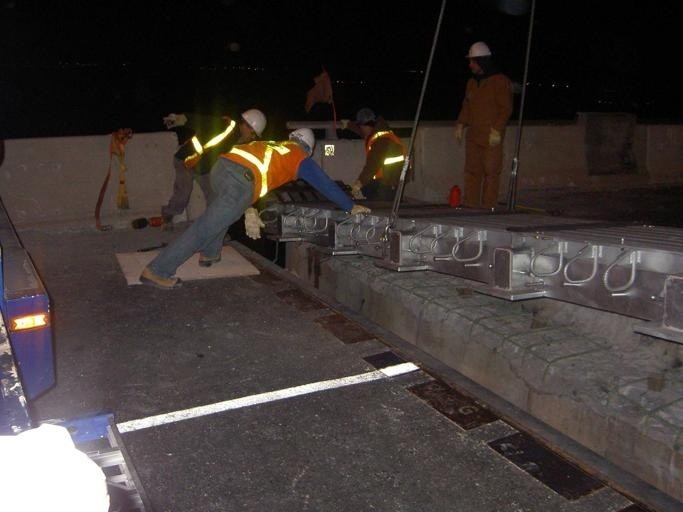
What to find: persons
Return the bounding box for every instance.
[160,109,267,243]
[139,128,371,291]
[340,108,407,202]
[454,42,513,209]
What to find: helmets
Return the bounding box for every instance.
[466,41,493,58]
[242,108,267,139]
[289,127,316,157]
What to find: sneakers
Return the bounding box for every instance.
[140,266,184,291]
[198,246,222,268]
[160,207,177,233]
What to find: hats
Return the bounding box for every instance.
[354,108,376,127]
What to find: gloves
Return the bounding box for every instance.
[351,180,368,200]
[339,119,352,130]
[163,113,188,130]
[242,207,267,241]
[489,127,502,148]
[345,203,373,216]
[454,123,464,145]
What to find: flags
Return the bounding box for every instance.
[302,74,335,113]
